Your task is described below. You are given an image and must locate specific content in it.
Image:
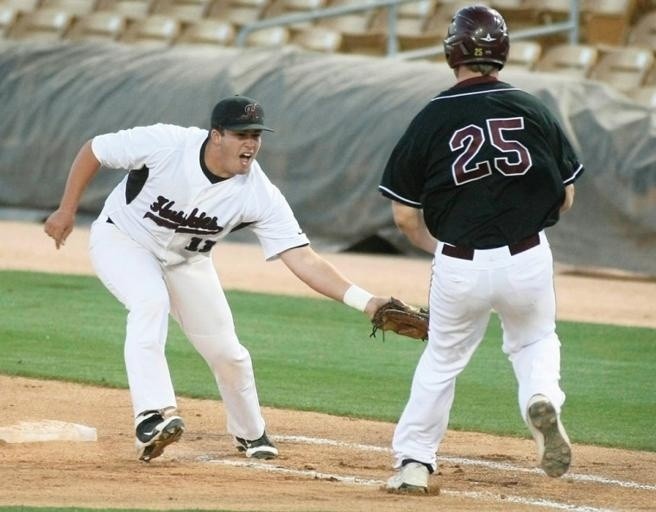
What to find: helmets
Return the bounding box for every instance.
[444,7,509,71]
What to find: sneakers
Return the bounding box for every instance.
[528,395,571,477]
[134,410,185,460]
[234,431,278,459]
[385,462,429,493]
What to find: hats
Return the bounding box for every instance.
[211,96,274,133]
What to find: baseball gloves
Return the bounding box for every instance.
[371,297,429,339]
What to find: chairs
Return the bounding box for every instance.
[0,0,655,87]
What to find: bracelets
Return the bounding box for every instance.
[343,284,374,312]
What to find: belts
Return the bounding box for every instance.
[442,233,540,260]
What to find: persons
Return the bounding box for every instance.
[44,93,392,464]
[377,5,586,495]
